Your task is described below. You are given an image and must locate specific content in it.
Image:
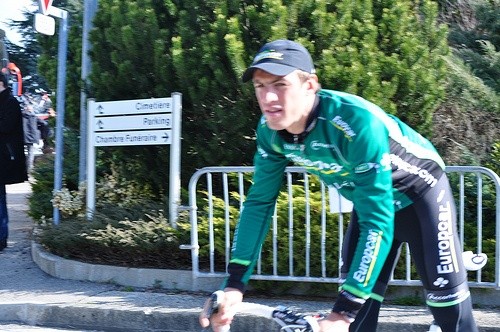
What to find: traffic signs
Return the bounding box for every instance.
[93,97,172,147]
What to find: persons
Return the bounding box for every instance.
[200,39,478,332]
[0,69,55,253]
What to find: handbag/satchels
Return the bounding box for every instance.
[4,151,28,184]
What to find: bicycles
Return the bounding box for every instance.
[197,251,488,332]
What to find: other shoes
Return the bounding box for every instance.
[0,243,7,254]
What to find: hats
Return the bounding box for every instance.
[242,40,316,83]
